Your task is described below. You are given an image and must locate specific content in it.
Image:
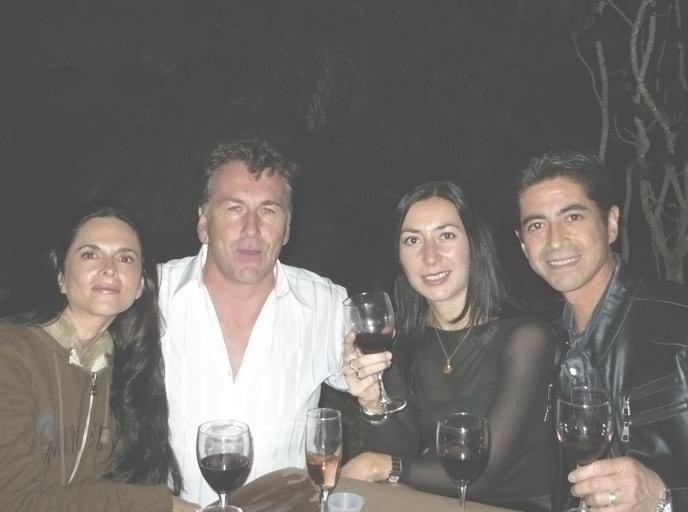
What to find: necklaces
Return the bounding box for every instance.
[431,316,473,374]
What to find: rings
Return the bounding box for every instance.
[350,359,357,370]
[609,491,616,505]
[356,368,362,380]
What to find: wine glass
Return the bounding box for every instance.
[340,289,409,417]
[555,385,617,512]
[435,411,493,512]
[304,408,343,510]
[194,421,253,512]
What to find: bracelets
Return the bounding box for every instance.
[357,405,389,426]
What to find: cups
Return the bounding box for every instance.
[326,493,365,512]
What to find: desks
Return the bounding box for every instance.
[187,466,527,512]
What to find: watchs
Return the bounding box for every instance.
[386,455,402,484]
[656,489,672,512]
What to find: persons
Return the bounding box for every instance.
[339,182,557,512]
[0,206,200,511]
[156,136,361,512]
[512,148,688,512]
